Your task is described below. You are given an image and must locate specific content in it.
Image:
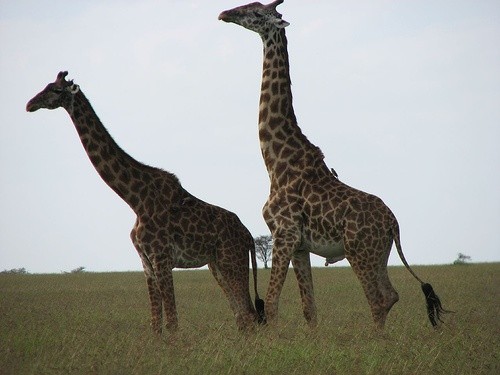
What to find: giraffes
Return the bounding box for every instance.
[26,70,267,335]
[218,0,457,331]
